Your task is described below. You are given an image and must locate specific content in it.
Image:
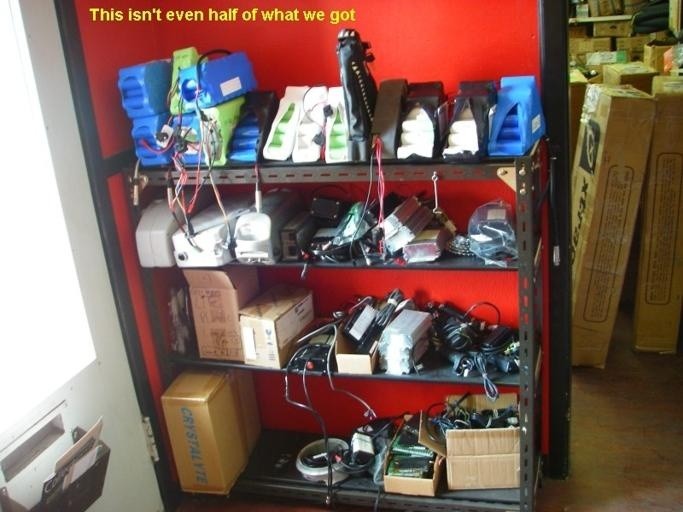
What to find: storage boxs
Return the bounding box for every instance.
[157,267,312,498]
[381,392,522,499]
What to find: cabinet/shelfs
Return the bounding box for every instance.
[52,1,571,510]
